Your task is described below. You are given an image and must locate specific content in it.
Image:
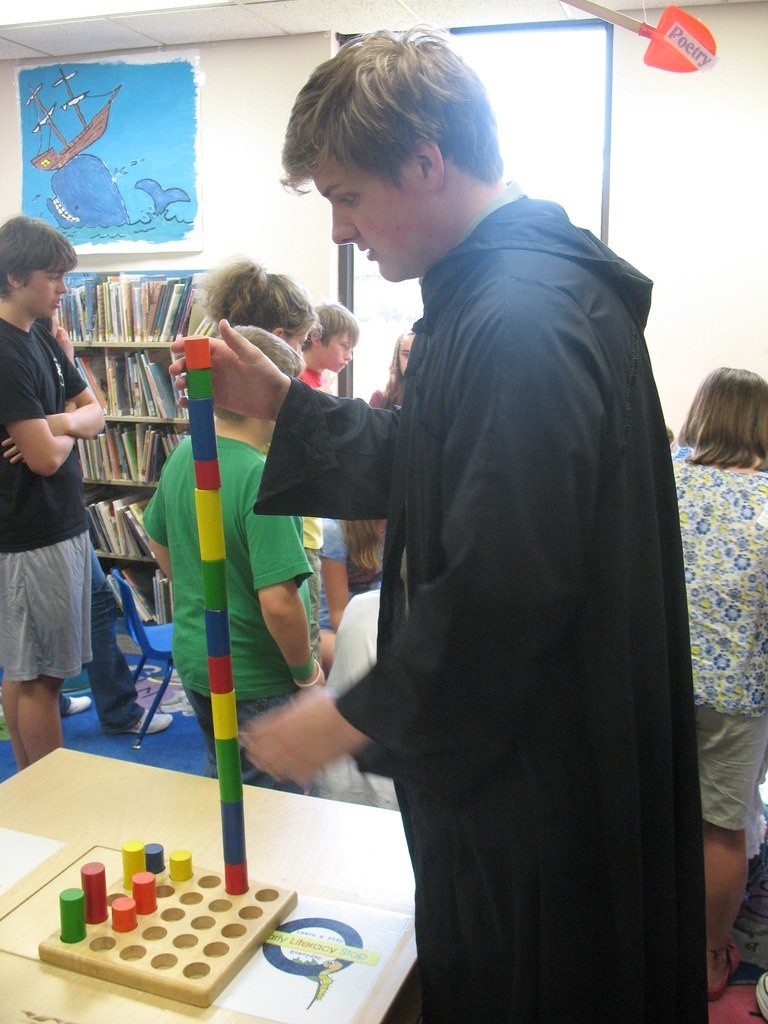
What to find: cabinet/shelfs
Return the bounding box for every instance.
[52,266,220,628]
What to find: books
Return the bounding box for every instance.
[58,274,213,626]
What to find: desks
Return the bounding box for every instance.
[0,747,422,1024]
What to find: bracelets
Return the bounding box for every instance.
[291,659,321,688]
[287,656,315,680]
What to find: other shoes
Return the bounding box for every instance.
[754,971,768,1023]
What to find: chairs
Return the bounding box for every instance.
[112,569,176,749]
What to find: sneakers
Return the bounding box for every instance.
[63,695,92,714]
[121,708,174,735]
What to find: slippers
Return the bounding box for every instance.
[706,938,740,999]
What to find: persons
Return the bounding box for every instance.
[199,258,361,664]
[0,215,173,770]
[670,367,768,1001]
[321,518,388,673]
[141,325,327,796]
[367,332,416,408]
[168,30,709,1024]
